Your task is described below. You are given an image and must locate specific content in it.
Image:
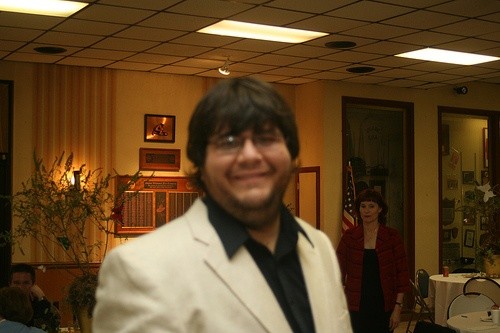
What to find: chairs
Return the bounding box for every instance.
[405,268,500,333]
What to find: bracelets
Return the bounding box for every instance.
[395,302,404,307]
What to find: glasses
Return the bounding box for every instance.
[216,134,283,148]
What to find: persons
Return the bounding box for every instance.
[336,189,410,333]
[92,76,353,333]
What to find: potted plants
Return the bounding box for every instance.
[474,246,500,277]
[0,148,154,333]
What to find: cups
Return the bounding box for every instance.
[491,310,500,324]
[443,266,448,276]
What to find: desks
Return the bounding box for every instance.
[428,273,500,327]
[446,311,500,333]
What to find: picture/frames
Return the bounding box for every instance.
[438,124,490,249]
[144,114,176,143]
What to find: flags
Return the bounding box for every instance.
[340,166,357,283]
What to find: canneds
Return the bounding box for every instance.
[443,266,449,277]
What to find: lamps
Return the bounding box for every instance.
[219,58,231,75]
[454,86,468,96]
